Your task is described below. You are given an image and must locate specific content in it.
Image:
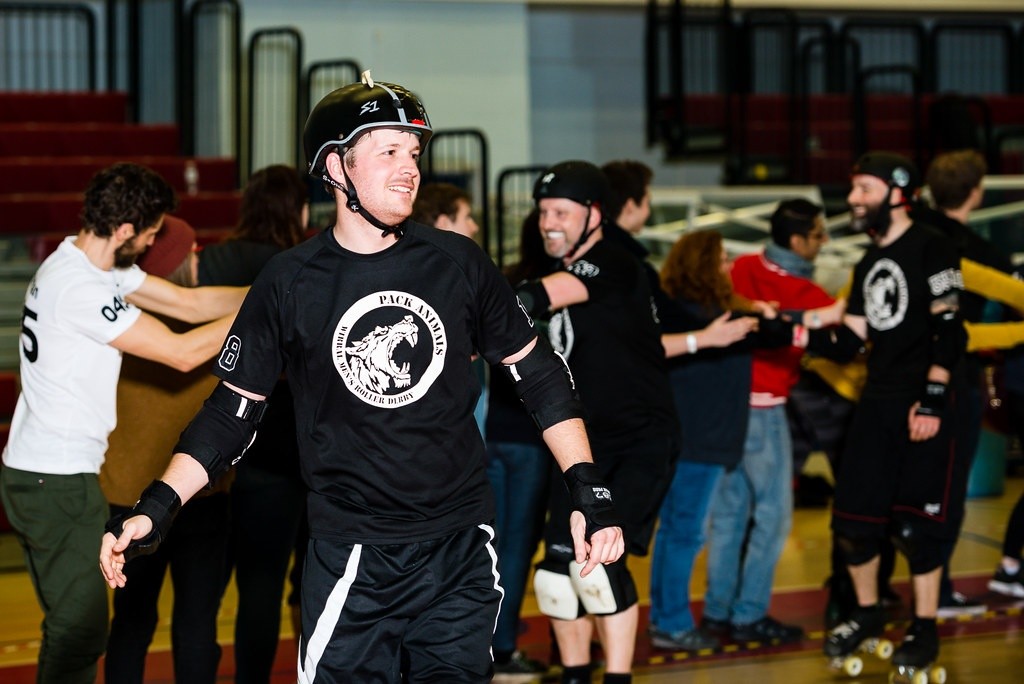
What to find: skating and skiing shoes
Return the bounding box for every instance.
[698,616,733,636]
[888,615,947,684]
[988,562,1024,618]
[722,615,803,653]
[824,604,894,677]
[933,580,987,625]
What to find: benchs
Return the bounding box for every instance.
[0,88,248,262]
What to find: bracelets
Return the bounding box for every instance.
[793,326,805,348]
[811,309,821,329]
[685,331,697,353]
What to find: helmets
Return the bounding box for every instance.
[303,70,434,178]
[533,160,615,225]
[848,148,916,204]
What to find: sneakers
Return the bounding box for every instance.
[649,628,718,664]
[492,651,546,683]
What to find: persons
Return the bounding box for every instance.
[0,147,1024,684]
[99,68,624,684]
